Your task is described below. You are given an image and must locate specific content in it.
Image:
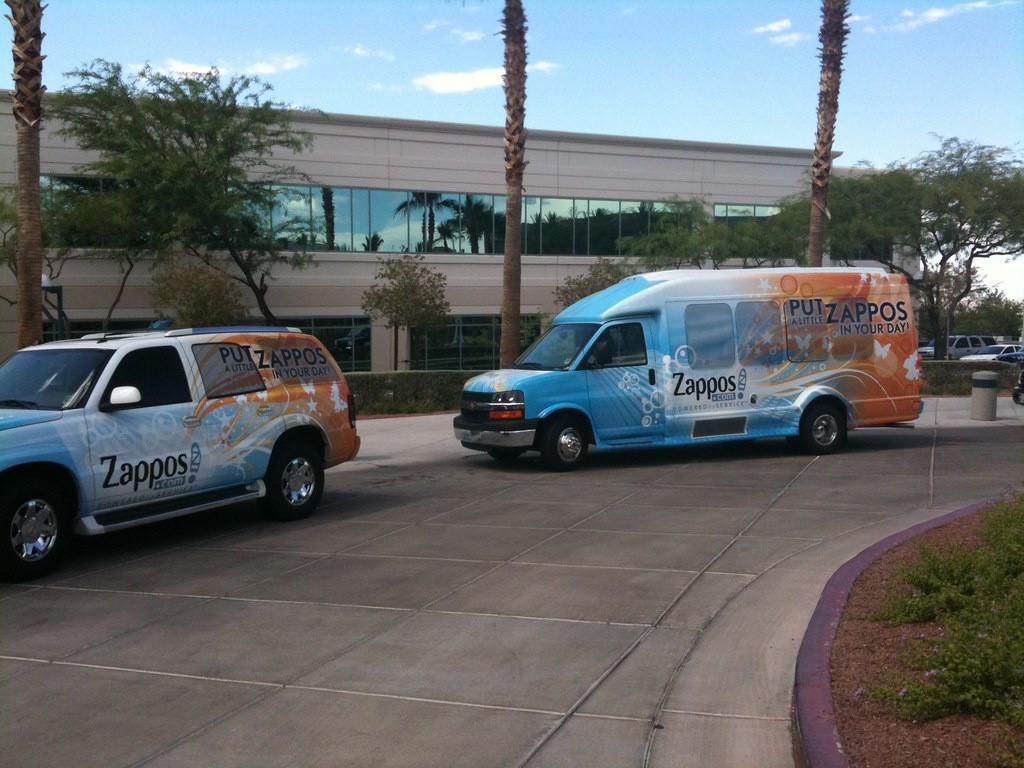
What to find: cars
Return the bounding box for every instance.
[996,346,1024,364]
[958,344,1022,362]
[919,339,935,358]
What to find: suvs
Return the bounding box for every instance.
[0,324,361,584]
[947,334,997,361]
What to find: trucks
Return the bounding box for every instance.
[454,266,925,472]
